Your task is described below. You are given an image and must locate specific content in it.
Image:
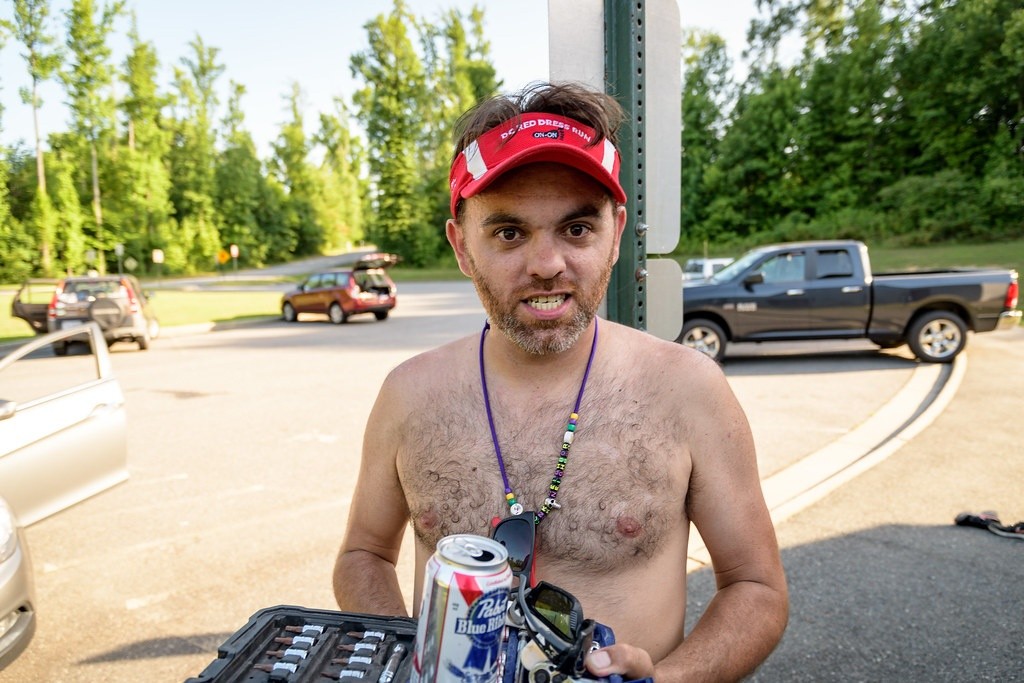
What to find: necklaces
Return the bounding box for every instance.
[479,315,597,526]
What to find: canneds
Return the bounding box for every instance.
[410,533,513,683]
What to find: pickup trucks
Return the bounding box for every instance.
[674,237,1022,364]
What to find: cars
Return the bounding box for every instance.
[0,322,134,670]
[683,258,733,279]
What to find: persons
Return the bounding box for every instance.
[331,79,789,683]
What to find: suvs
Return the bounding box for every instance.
[280,253,404,324]
[9,273,161,357]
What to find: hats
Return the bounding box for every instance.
[450,113,628,220]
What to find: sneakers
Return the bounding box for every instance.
[954,510,1024,539]
